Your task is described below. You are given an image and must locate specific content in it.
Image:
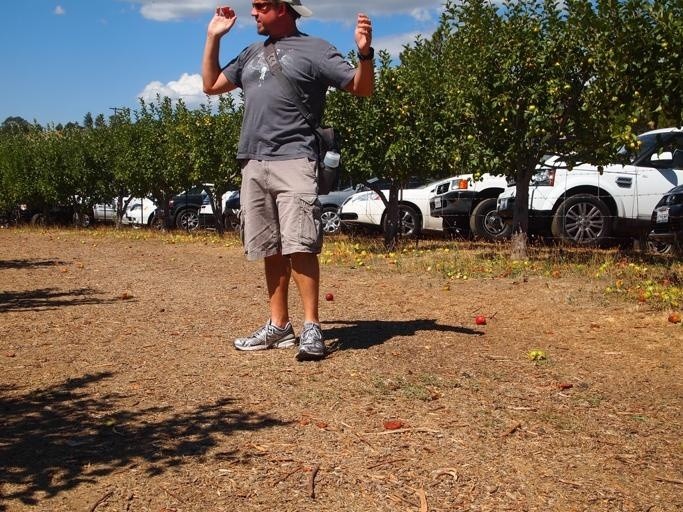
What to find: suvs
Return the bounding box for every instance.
[496,125,683,248]
[428,149,583,243]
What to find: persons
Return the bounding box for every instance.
[199,1,375,362]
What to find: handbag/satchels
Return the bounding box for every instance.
[313,124,342,195]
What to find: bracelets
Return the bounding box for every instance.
[357,47,374,61]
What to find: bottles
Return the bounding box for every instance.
[323,149,340,168]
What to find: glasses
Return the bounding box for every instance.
[252,2,283,14]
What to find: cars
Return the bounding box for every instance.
[318,174,425,236]
[336,171,508,239]
[67,183,242,230]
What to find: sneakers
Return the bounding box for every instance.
[233,317,297,351]
[293,320,327,359]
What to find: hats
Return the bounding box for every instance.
[283,0,313,18]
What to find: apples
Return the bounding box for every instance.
[320,27,682,188]
[530,352,537,358]
[476,316,486,325]
[669,313,681,324]
[320,237,683,310]
[537,351,544,355]
